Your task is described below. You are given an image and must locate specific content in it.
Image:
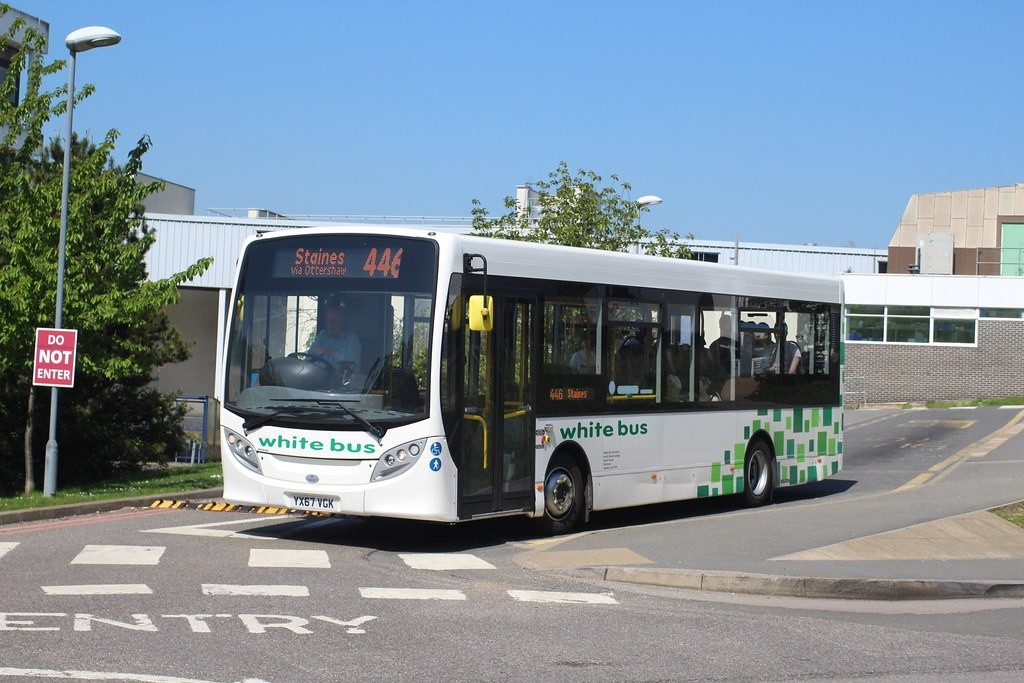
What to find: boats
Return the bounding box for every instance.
[634,194,663,253]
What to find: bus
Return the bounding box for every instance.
[219,222,846,537]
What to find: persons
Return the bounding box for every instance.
[698,377,718,402]
[305,305,362,378]
[763,322,802,374]
[570,331,597,375]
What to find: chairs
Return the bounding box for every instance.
[616,336,644,387]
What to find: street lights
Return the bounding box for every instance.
[44,26,122,492]
